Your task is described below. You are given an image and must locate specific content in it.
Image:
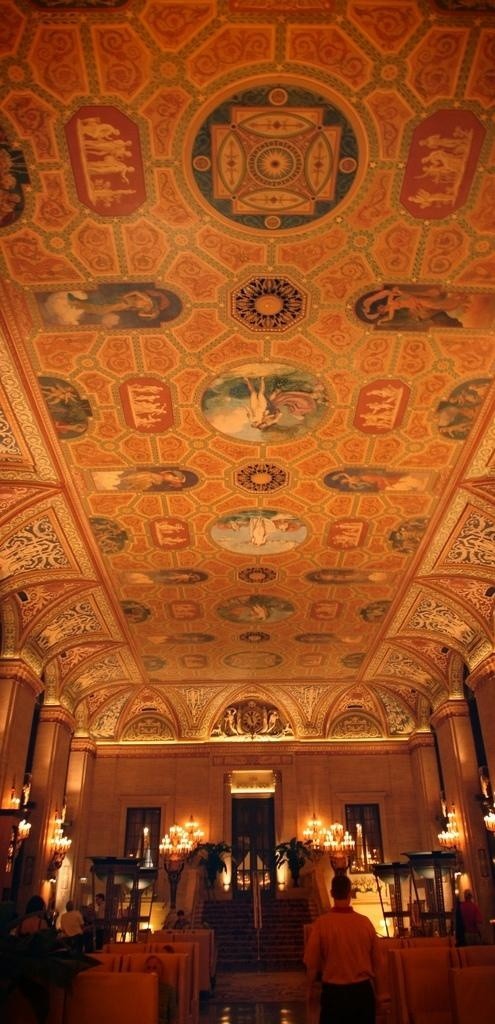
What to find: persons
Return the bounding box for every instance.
[303,875,384,1024]
[451,888,484,944]
[0,889,209,1023]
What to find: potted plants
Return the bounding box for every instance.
[277,837,309,906]
[197,841,229,906]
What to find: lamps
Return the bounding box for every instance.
[158,816,210,911]
[301,814,356,872]
[437,807,460,848]
[44,814,72,881]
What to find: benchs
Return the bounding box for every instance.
[1,928,216,1024]
[371,934,494,1022]
